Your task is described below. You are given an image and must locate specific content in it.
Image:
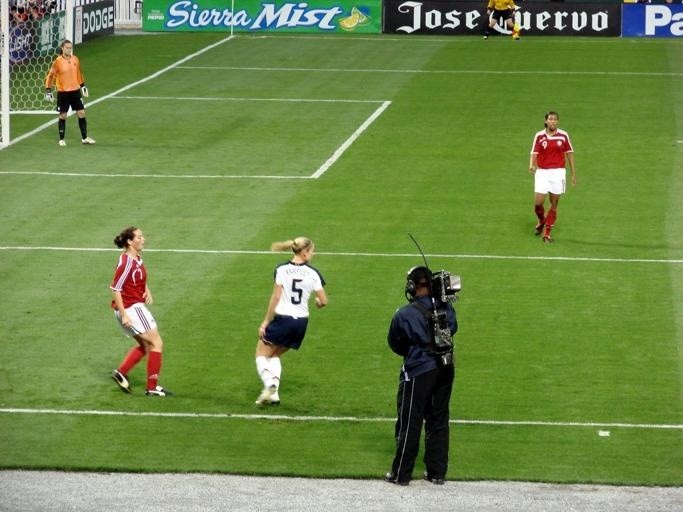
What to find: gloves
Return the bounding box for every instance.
[82,86,88,97]
[46,93,54,103]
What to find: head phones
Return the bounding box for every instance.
[404,265,425,297]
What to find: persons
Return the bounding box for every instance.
[483,0,522,42]
[253,236,329,407]
[528,109,576,244]
[9,0,56,25]
[382,264,458,487]
[43,37,96,147]
[109,225,177,399]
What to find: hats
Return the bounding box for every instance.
[407,266,431,282]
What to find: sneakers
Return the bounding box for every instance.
[265,388,280,405]
[535,216,546,235]
[385,473,410,486]
[423,471,445,485]
[111,369,134,393]
[145,385,173,397]
[82,137,95,144]
[58,138,66,147]
[543,228,551,242]
[255,382,277,409]
